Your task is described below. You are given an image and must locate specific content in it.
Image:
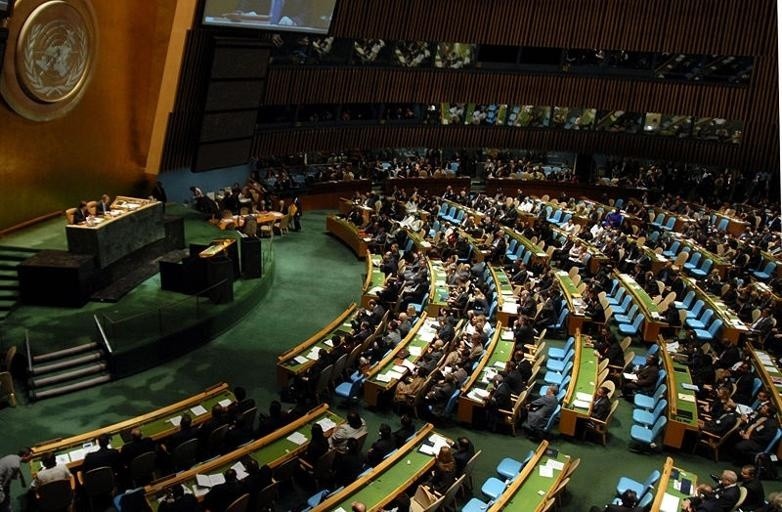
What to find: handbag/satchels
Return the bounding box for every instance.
[414,485,436,509]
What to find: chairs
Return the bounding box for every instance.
[21,141,782,511]
[0,346,17,406]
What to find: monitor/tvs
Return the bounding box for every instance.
[199,0,340,38]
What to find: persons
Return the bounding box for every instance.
[96,194,112,215]
[256,146,749,183]
[0,180,781,511]
[73,199,90,224]
[754,166,771,196]
